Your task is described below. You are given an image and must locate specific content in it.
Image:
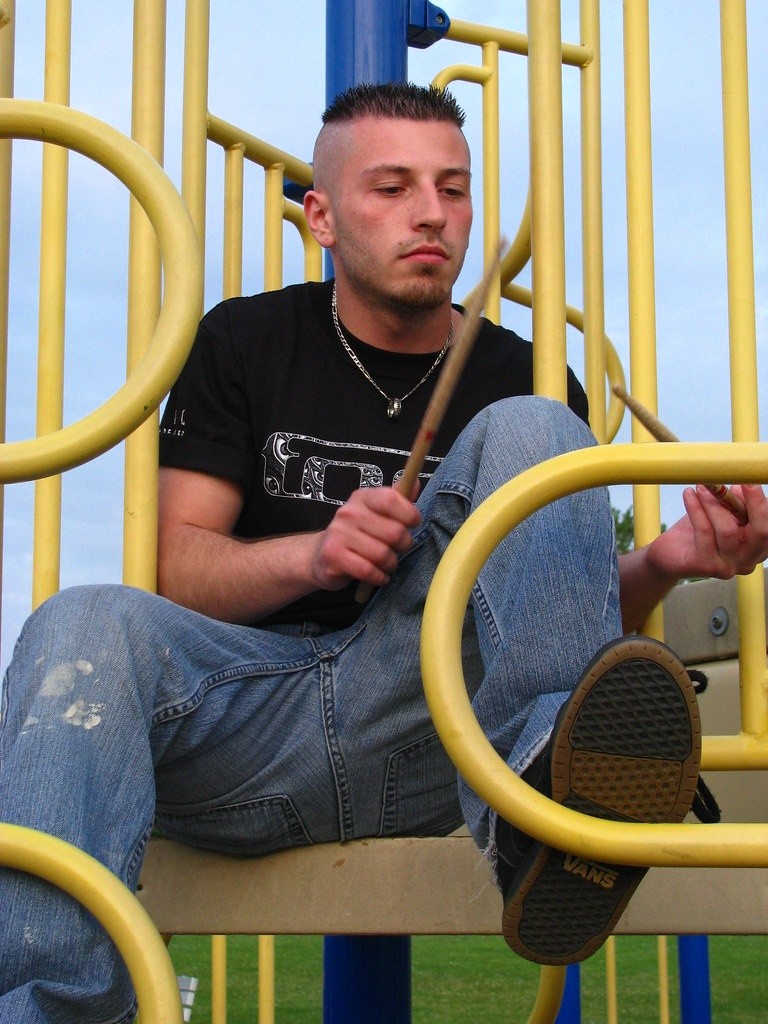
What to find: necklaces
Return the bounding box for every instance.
[332,283,453,416]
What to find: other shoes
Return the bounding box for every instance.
[495,635,722,967]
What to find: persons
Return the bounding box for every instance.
[1,83,768,1024]
